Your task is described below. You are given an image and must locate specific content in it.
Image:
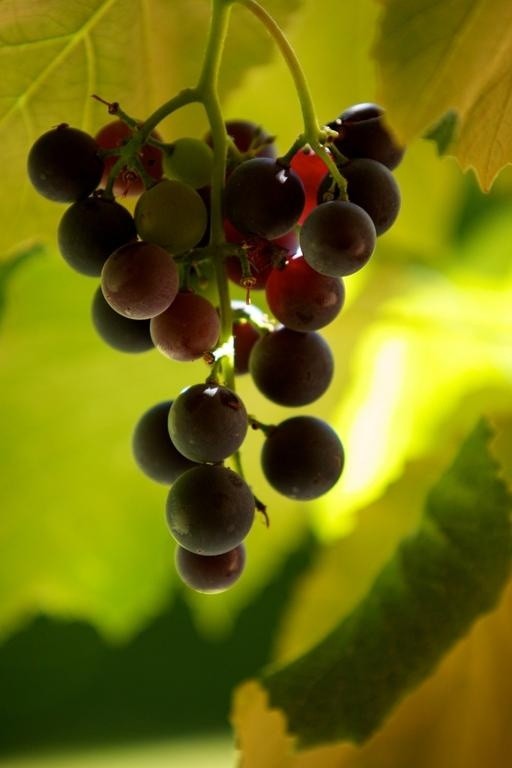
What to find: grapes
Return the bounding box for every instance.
[26,102,408,595]
